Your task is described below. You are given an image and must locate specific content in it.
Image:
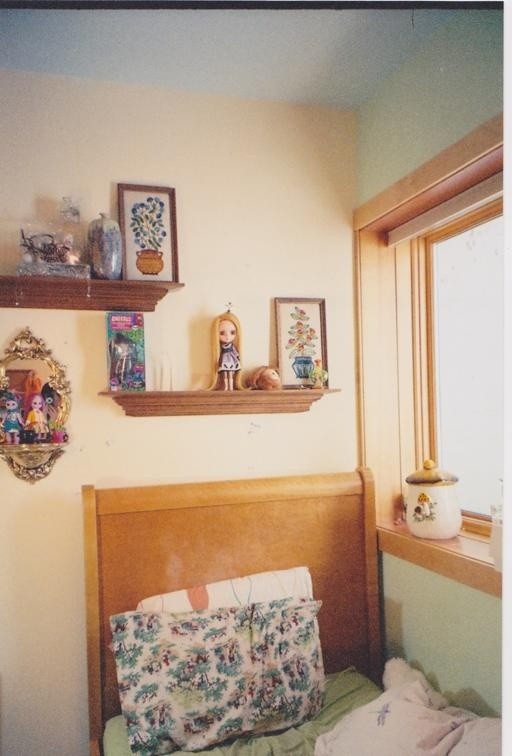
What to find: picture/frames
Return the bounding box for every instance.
[274,297,328,390]
[117,184,179,283]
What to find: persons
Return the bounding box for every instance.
[211,313,280,392]
[0,388,62,444]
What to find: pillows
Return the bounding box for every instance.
[135,565,314,614]
[111,598,323,754]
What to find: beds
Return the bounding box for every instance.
[81,468,502,756]
